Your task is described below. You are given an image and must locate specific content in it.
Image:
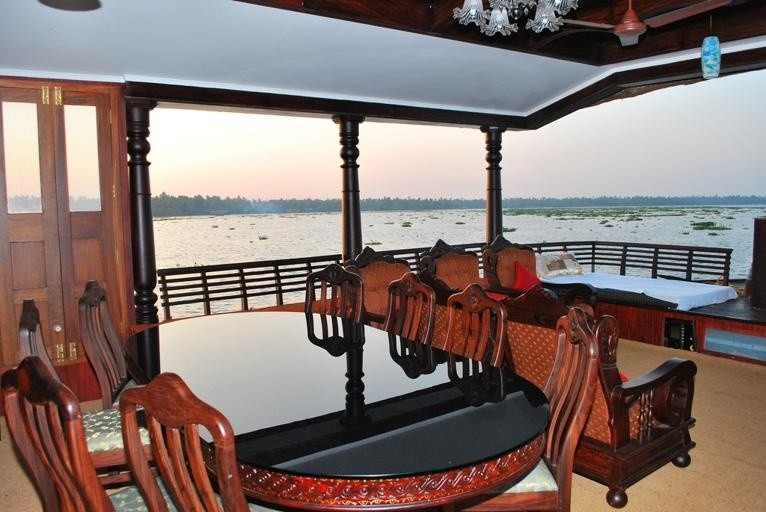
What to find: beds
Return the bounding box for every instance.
[536,270,738,312]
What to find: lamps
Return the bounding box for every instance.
[449,1,721,81]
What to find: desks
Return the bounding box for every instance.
[120,307,553,512]
